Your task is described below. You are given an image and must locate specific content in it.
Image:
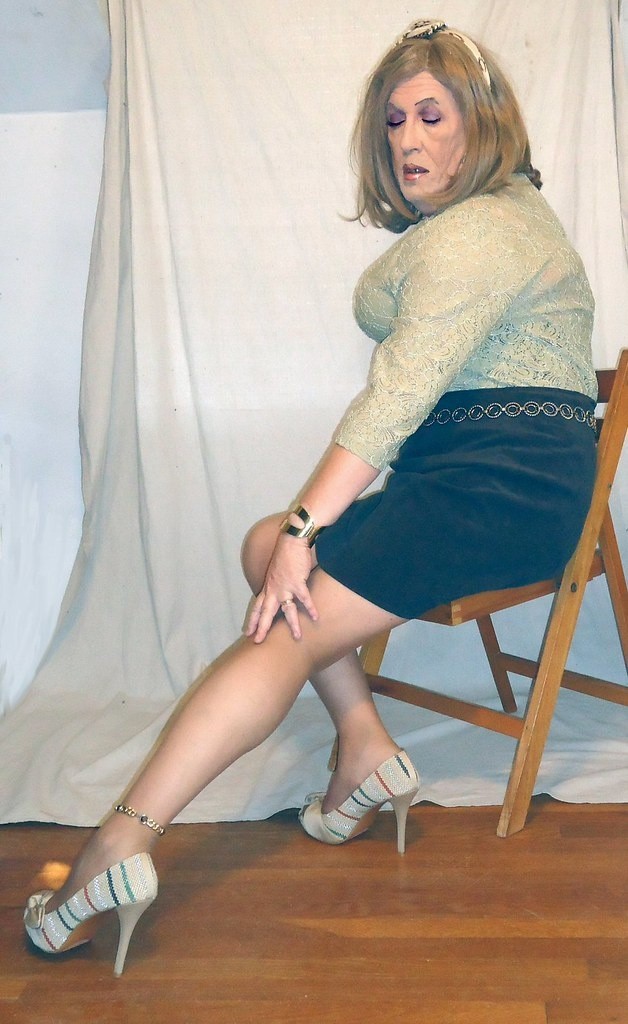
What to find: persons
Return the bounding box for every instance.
[23,18,599,986]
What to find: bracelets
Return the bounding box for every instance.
[280,503,328,548]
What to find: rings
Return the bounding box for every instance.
[281,598,295,605]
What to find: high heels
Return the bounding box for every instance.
[297,748,420,852]
[22,852,158,977]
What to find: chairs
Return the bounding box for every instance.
[327,349,628,838]
